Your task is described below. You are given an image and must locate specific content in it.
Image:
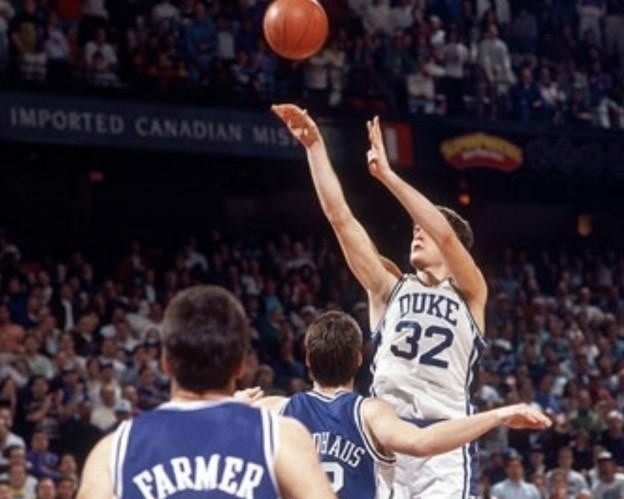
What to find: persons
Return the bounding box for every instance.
[0,0,624,499]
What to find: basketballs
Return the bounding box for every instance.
[263,0,329,60]
